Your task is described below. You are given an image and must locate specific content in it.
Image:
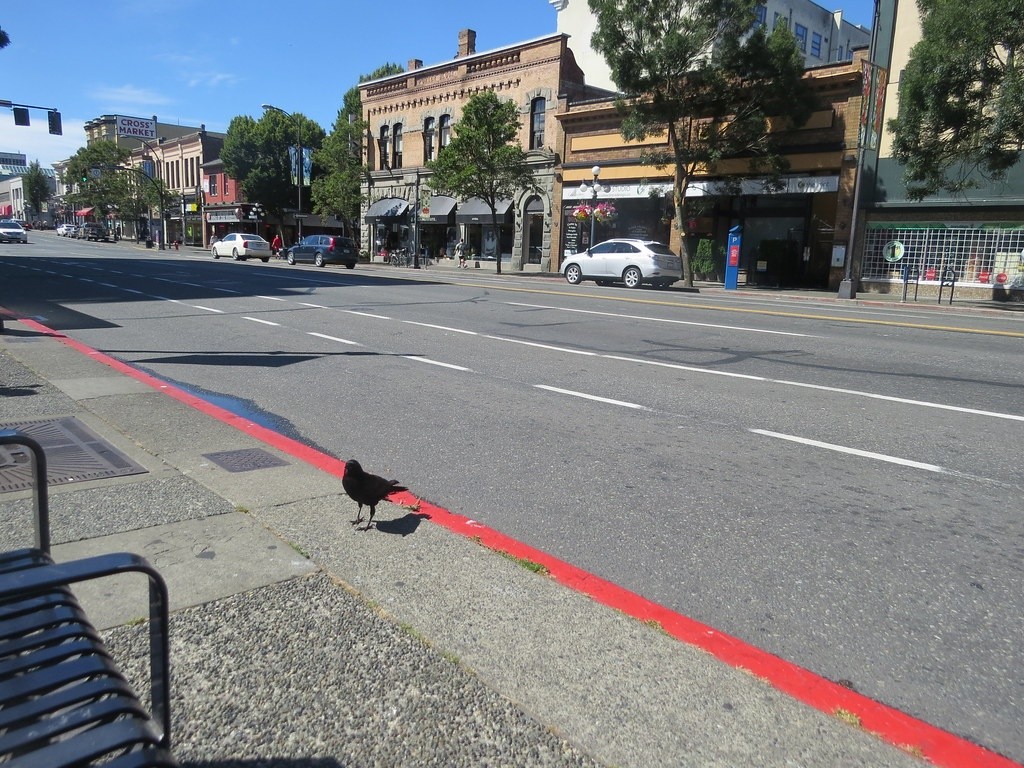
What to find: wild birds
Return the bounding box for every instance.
[341,459,409,533]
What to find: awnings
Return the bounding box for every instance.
[75,207,94,216]
[365,198,409,224]
[456,196,514,224]
[419,195,457,224]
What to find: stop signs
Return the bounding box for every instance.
[978,273,988,283]
[925,270,935,280]
[996,273,1007,282]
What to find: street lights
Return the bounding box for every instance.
[120,134,164,250]
[250,203,265,236]
[107,203,120,240]
[261,104,302,244]
[580,166,611,248]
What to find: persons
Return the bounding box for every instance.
[117,224,120,236]
[272,234,281,259]
[210,232,218,245]
[454,238,467,268]
[485,230,496,261]
[55,218,58,228]
[32,220,48,229]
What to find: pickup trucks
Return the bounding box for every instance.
[77,221,110,242]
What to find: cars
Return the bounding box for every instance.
[11,219,28,231]
[67,226,79,238]
[287,235,359,269]
[211,232,273,262]
[0,222,27,244]
[56,223,75,237]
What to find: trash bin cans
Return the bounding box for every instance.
[146,240,153,248]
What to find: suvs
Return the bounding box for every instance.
[560,237,682,289]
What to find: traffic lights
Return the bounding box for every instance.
[81,167,87,182]
